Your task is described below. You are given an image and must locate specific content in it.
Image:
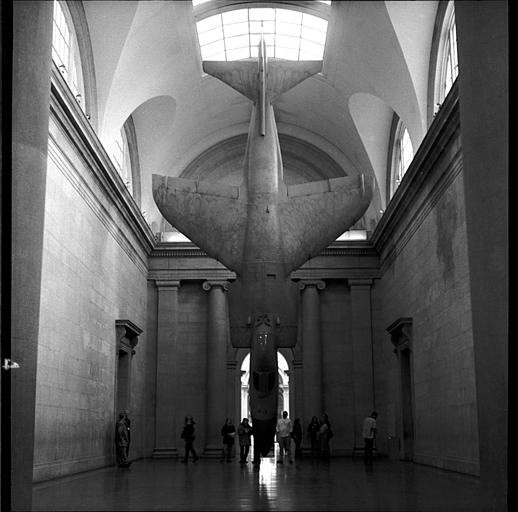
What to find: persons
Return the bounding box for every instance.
[238,419,252,463]
[221,419,236,464]
[292,418,302,460]
[276,411,293,463]
[317,412,329,462]
[116,410,131,467]
[363,412,378,472]
[308,415,319,455]
[181,417,198,463]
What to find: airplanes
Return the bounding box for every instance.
[151,39,376,458]
